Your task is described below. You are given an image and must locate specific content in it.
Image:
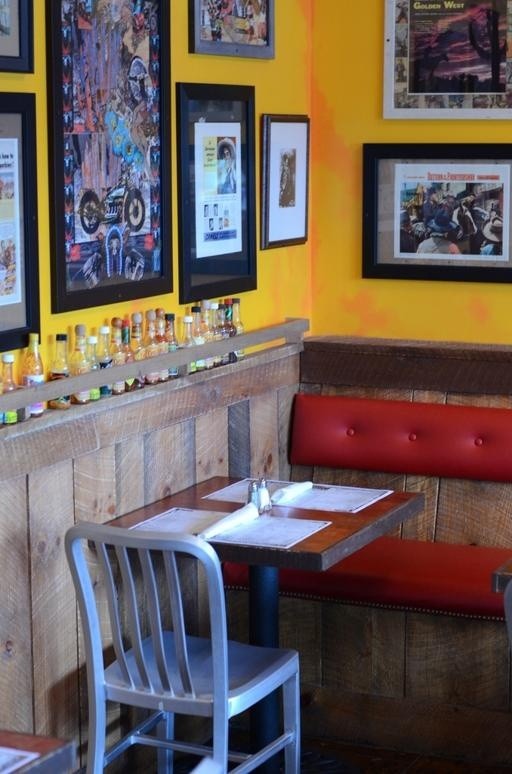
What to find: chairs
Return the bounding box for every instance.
[57,520,302,773]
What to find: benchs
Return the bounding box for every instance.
[204,388,510,623]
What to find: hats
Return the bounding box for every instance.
[217,138,236,160]
[481,215,503,242]
[427,210,459,233]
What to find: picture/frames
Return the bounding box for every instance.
[380,0,511,123]
[360,140,511,281]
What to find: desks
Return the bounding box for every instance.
[2,726,78,773]
[81,470,425,774]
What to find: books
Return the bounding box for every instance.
[127,504,331,553]
[200,470,395,519]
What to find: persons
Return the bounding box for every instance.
[216,136,238,193]
[399,184,502,254]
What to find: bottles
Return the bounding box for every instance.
[260,478,272,513]
[248,481,262,513]
[2,300,246,421]
